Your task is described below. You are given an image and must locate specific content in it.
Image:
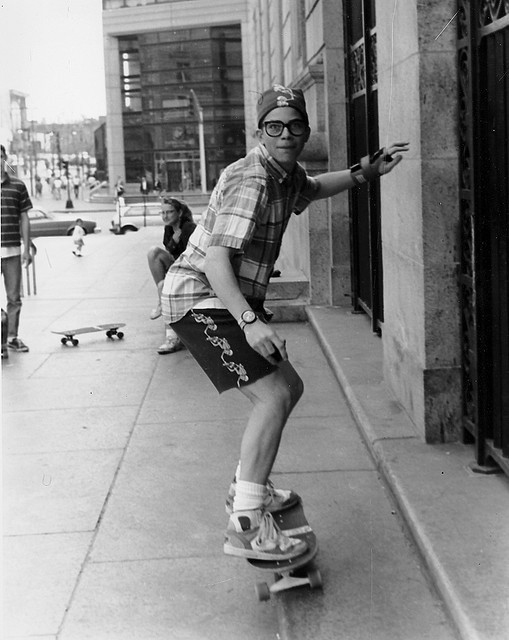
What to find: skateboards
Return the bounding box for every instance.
[1,309,8,358]
[51,322,126,346]
[244,491,323,602]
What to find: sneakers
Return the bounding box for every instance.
[150,302,162,320]
[222,506,307,560]
[156,335,183,354]
[224,476,298,516]
[7,338,29,353]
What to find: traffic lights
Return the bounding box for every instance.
[63,161,68,169]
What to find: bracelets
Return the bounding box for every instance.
[347,160,367,185]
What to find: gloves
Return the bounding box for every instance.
[350,146,392,186]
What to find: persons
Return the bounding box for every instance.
[161,86,410,560]
[115,190,125,219]
[114,176,124,197]
[1,144,33,357]
[238,310,257,329]
[34,158,108,199]
[140,175,150,196]
[72,218,86,256]
[147,197,198,354]
[153,179,161,193]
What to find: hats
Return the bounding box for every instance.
[255,86,309,128]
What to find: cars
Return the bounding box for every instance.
[19,210,96,236]
[110,204,203,234]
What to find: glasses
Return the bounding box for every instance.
[259,119,307,138]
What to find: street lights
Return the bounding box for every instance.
[189,90,207,195]
